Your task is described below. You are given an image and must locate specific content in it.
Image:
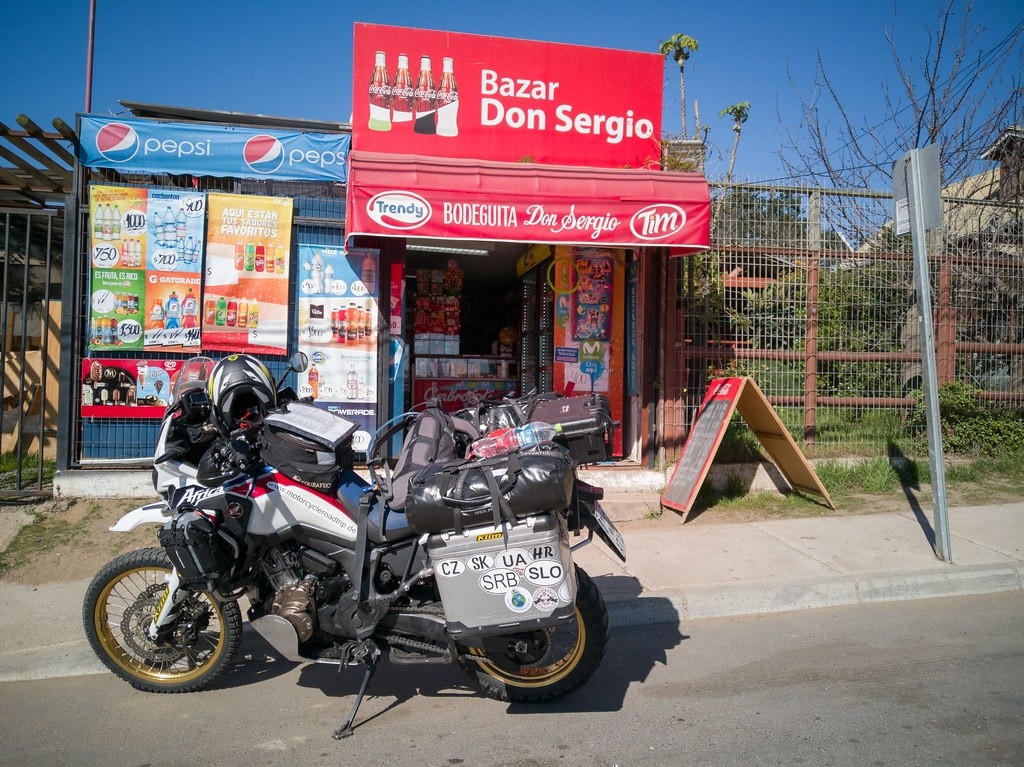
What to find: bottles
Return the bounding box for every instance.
[151,298,164,329]
[362,253,375,294]
[331,303,372,347]
[166,290,181,329]
[255,240,265,272]
[181,287,198,329]
[205,293,258,328]
[308,363,324,399]
[91,315,118,345]
[234,239,244,270]
[245,240,254,271]
[312,251,334,295]
[154,205,201,264]
[347,364,365,399]
[367,50,458,137]
[266,242,285,273]
[122,239,142,267]
[94,203,120,241]
[115,293,139,315]
[472,421,563,461]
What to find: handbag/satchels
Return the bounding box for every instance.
[405,441,576,534]
[260,400,354,493]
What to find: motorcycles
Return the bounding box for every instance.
[82,349,633,742]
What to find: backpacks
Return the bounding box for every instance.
[370,401,481,542]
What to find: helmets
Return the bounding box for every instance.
[209,354,277,427]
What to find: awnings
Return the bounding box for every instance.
[341,152,713,256]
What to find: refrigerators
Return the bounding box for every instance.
[516,244,625,460]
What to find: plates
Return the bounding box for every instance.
[577,307,586,315]
[577,326,591,338]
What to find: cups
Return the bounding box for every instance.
[596,291,600,300]
